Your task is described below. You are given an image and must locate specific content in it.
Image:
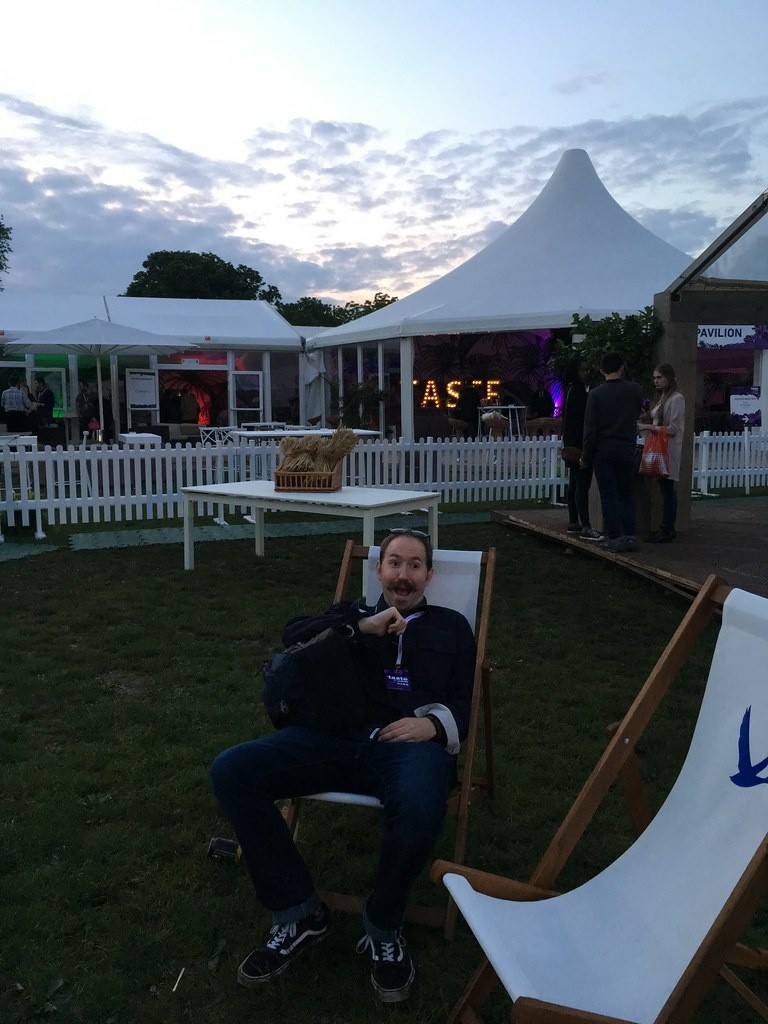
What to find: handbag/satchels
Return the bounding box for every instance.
[640,426,671,477]
[561,447,581,462]
[259,599,364,730]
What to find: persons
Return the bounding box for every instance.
[75,383,115,443]
[455,385,516,438]
[530,383,554,418]
[579,353,643,550]
[1,376,55,451]
[636,364,685,542]
[181,390,199,423]
[210,530,476,1001]
[561,357,605,541]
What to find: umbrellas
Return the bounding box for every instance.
[2,315,199,431]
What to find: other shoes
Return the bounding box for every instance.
[624,537,636,549]
[600,540,620,552]
[648,526,676,540]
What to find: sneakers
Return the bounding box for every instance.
[237,902,333,984]
[568,526,583,533]
[580,529,604,541]
[357,925,415,1003]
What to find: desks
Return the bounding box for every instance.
[230,429,384,481]
[180,480,441,569]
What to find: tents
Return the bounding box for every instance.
[305,149,768,445]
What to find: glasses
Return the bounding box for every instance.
[387,528,431,543]
[578,368,590,372]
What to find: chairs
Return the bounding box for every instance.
[199,425,315,446]
[279,539,496,940]
[431,572,768,1024]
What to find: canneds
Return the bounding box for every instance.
[207,836,242,864]
